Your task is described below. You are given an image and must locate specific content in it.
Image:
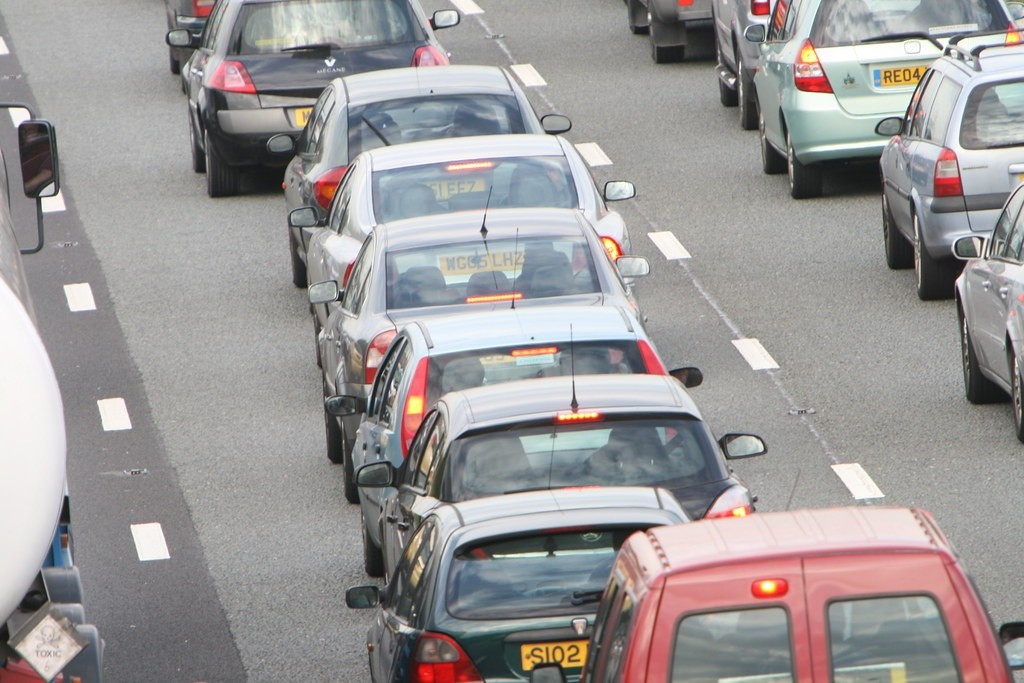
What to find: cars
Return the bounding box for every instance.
[740,0,1024,201]
[349,374,771,621]
[952,180,1024,446]
[574,502,1024,682]
[344,480,702,683]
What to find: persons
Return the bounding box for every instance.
[19,124,54,194]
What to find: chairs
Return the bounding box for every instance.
[446,102,501,142]
[540,347,629,380]
[390,267,462,306]
[441,356,485,398]
[459,441,536,495]
[452,561,527,613]
[354,114,397,159]
[514,252,575,294]
[582,429,677,484]
[384,182,443,223]
[505,173,548,207]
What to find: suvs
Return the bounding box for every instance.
[617,0,721,63]
[710,0,783,132]
[875,28,1024,300]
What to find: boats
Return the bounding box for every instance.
[155,0,705,580]
